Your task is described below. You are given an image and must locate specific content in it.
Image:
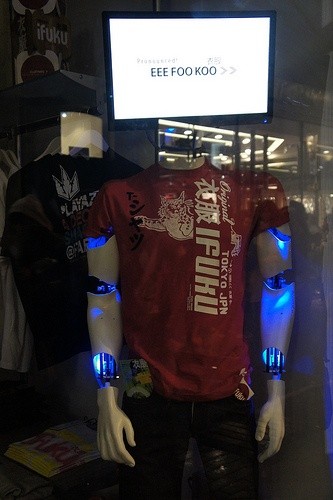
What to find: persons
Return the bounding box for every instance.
[85,144,295,500]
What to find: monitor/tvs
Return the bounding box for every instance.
[101,9,277,132]
